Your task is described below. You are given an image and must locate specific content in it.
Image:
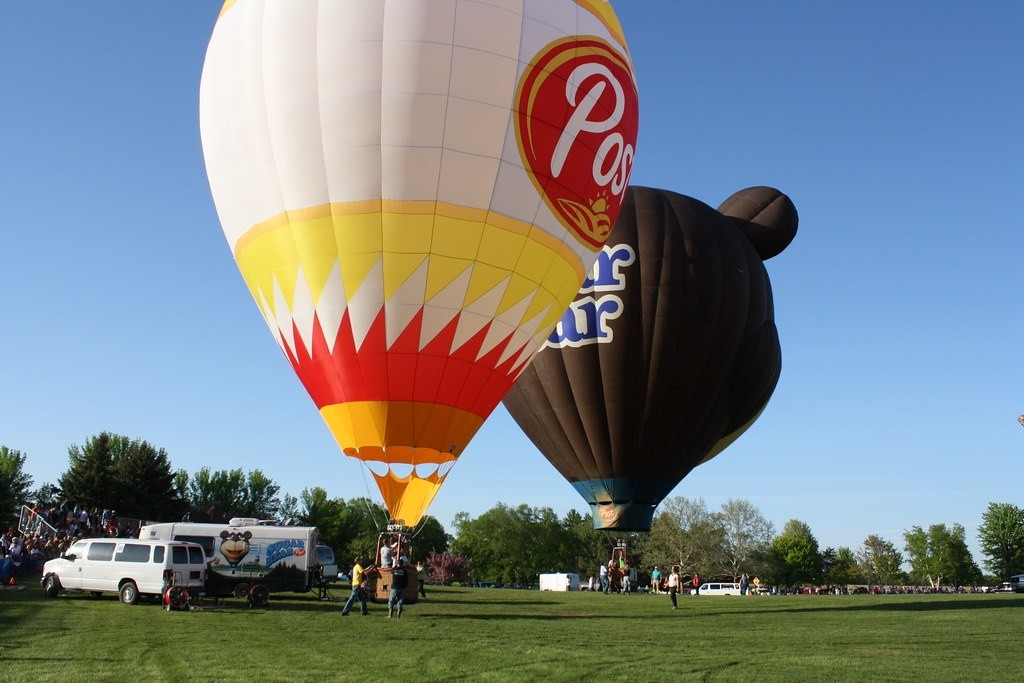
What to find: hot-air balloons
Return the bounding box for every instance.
[197,0,641,603]
[499,184,800,587]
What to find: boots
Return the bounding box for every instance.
[386,607,393,618]
[396,608,401,619]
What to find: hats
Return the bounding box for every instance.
[12,537,19,541]
[354,556,363,562]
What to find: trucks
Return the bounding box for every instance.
[139,517,317,604]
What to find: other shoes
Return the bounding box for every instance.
[673,606,678,609]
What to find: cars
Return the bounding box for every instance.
[991,582,1016,594]
[1010,575,1024,593]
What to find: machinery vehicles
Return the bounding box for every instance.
[598,539,638,592]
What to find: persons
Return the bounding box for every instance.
[786,584,844,595]
[664,566,680,609]
[651,566,662,594]
[380,539,393,568]
[873,585,966,594]
[0,497,139,585]
[600,549,631,595]
[693,574,702,595]
[740,573,749,595]
[416,561,426,599]
[393,547,408,567]
[341,556,373,616]
[387,559,408,619]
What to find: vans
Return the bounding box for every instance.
[316,546,338,582]
[41,538,206,605]
[690,583,748,596]
